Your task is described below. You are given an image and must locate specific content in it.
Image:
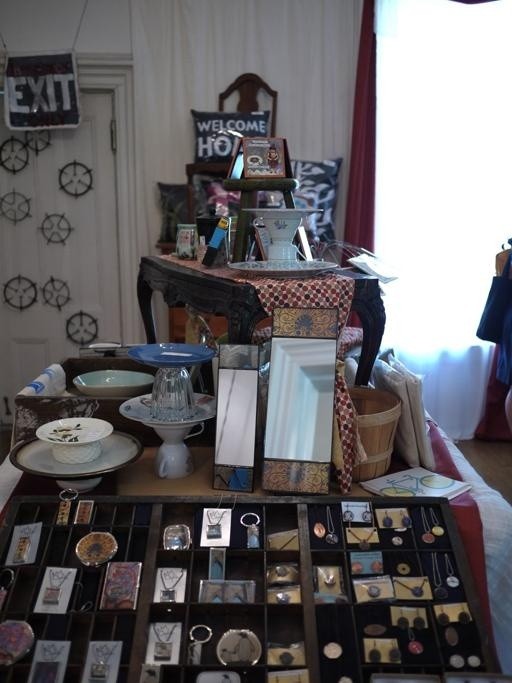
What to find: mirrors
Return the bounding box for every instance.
[213,367,258,469]
[262,336,336,464]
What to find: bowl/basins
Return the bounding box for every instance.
[51,475,105,496]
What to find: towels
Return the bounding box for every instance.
[14,363,73,398]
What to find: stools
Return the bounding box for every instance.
[136,245,386,390]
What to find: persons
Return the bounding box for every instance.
[266,145,280,169]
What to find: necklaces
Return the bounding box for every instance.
[392,577,429,598]
[158,569,184,604]
[150,623,177,662]
[430,553,450,603]
[444,555,461,589]
[427,505,445,539]
[31,644,64,682]
[344,528,378,551]
[203,493,239,542]
[322,505,340,548]
[419,505,436,547]
[41,570,71,606]
[89,641,120,681]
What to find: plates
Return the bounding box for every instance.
[73,369,156,398]
[241,207,323,218]
[119,391,217,427]
[9,428,144,481]
[35,417,114,446]
[226,259,341,279]
[127,342,214,367]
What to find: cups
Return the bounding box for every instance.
[151,367,193,418]
[154,443,192,479]
[268,242,311,269]
[175,223,201,262]
[255,218,305,243]
[142,423,205,442]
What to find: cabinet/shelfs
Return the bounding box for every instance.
[0,356,504,683]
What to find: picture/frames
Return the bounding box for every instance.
[242,135,287,178]
